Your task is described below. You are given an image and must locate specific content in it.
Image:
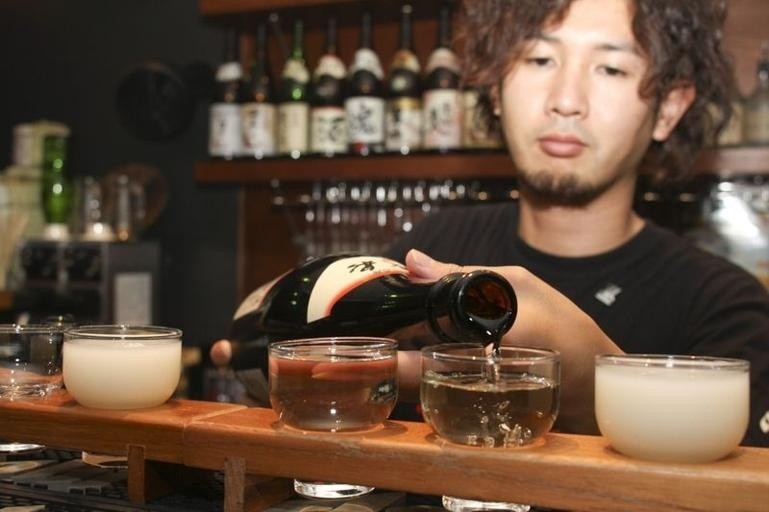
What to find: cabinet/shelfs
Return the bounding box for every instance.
[193,0,769,343]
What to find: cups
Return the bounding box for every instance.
[592,351,753,463]
[61,324,185,470]
[0,322,64,402]
[299,181,469,262]
[267,335,401,501]
[416,340,562,512]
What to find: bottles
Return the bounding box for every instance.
[712,43,769,149]
[307,3,347,157]
[239,11,275,158]
[273,3,309,157]
[0,118,72,306]
[346,4,387,156]
[463,84,490,147]
[42,134,71,241]
[207,10,243,157]
[421,3,462,151]
[227,253,518,410]
[382,3,421,153]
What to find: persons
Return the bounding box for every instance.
[212,0,768,449]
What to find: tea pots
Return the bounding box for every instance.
[71,176,148,244]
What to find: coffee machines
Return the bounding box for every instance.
[17,242,156,344]
